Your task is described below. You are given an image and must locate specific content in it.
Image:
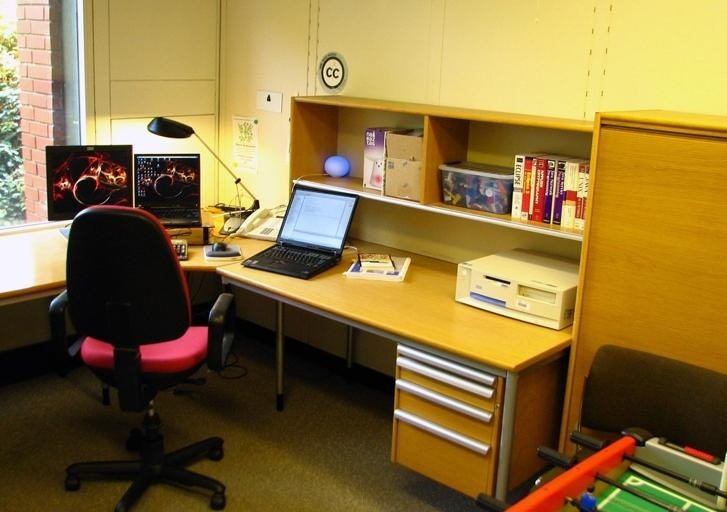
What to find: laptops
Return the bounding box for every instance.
[134,152,202,227]
[241,183,360,279]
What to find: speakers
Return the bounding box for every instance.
[219,215,246,235]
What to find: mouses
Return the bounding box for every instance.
[212,241,227,252]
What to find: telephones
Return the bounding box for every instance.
[236,208,286,242]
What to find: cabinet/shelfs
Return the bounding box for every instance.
[558,111,726,458]
[287,96,594,241]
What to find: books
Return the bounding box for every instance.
[511,152,591,229]
[348,256,411,281]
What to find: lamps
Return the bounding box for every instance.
[148,117,260,219]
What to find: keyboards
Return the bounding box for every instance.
[170,238,188,260]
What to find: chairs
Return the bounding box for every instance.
[51,206,226,512]
[578,342,724,464]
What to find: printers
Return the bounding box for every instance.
[453,248,580,330]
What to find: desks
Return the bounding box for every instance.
[217,238,572,510]
[0,206,278,307]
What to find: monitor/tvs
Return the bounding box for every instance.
[45,144,133,240]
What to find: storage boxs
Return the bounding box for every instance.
[438,160,514,214]
[384,128,421,202]
[362,126,391,190]
